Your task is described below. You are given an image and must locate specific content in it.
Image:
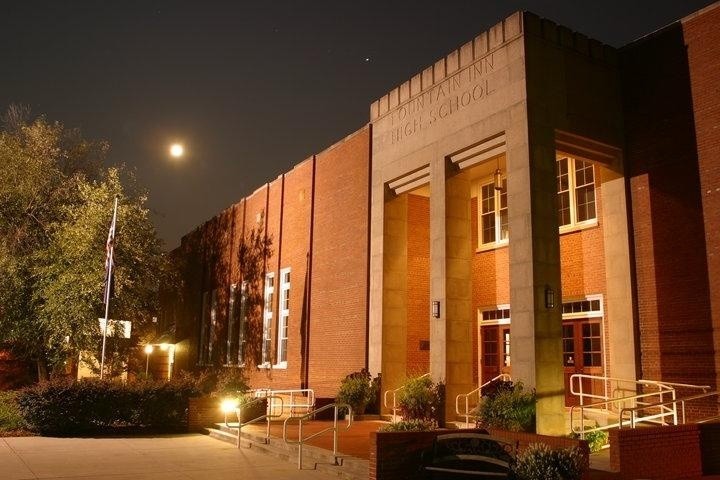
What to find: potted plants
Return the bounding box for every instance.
[334,368,382,417]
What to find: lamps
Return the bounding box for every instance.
[493,157,504,191]
[544,285,555,309]
[431,299,440,319]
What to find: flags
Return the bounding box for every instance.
[100,207,116,304]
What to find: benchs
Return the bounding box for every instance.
[416,433,520,480]
